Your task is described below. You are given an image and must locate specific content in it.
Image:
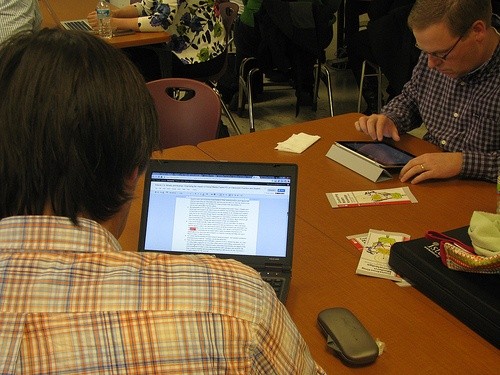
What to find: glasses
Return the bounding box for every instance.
[415,22,472,59]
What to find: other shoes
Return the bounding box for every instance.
[228,92,246,114]
[363,98,387,116]
[217,123,228,139]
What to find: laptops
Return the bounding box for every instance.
[138,159,298,306]
[43,0,99,35]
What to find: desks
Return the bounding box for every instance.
[117,113,500,375]
[38,0,172,48]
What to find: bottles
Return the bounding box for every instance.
[96,0,112,40]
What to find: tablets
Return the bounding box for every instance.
[333,140,417,167]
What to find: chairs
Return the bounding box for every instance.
[174,2,240,136]
[146,79,221,149]
[238,17,336,131]
[357,59,384,113]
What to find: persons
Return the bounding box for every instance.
[326,0,422,116]
[237,0,334,99]
[355,0,500,184]
[88,0,228,81]
[0,26,326,375]
